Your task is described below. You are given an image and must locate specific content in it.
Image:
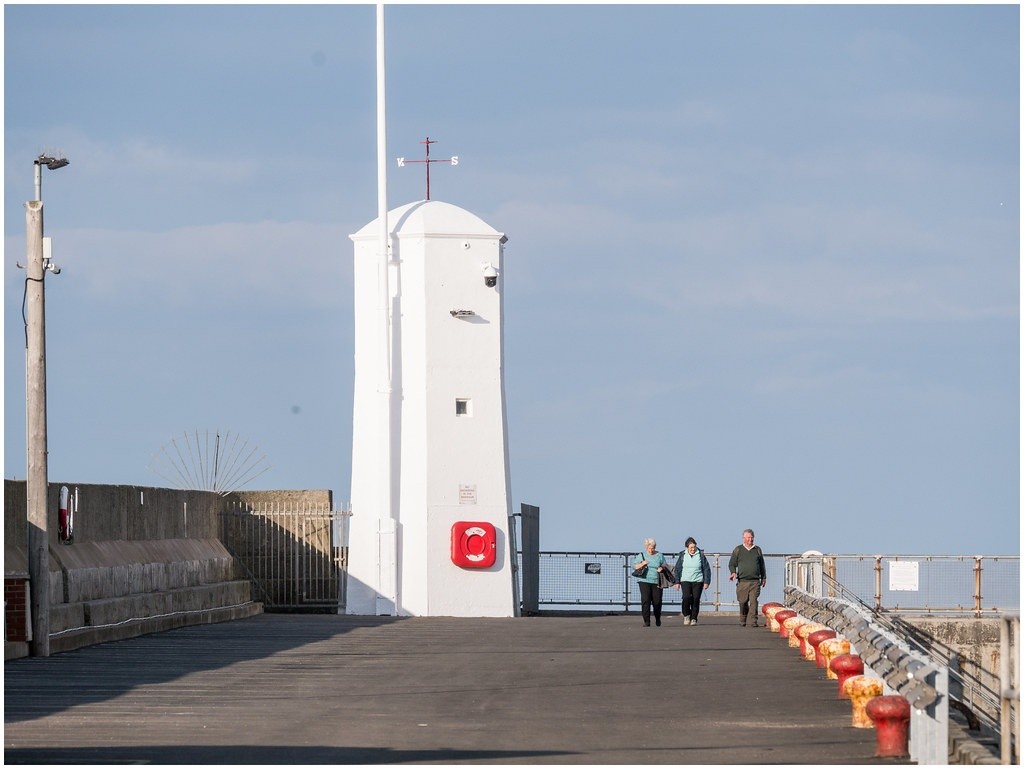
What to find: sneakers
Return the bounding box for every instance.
[751,624,758,627]
[740,621,746,627]
[683,615,690,625]
[690,619,697,625]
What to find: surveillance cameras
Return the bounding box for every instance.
[48,264,61,275]
[484,268,497,287]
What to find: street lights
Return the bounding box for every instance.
[20,152,70,656]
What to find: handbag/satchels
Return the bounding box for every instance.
[632,552,648,579]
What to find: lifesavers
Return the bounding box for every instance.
[945,698,978,736]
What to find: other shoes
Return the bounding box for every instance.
[643,622,650,627]
[655,616,661,627]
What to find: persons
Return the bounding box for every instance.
[675,537,711,626]
[632,538,666,627]
[728,529,767,627]
